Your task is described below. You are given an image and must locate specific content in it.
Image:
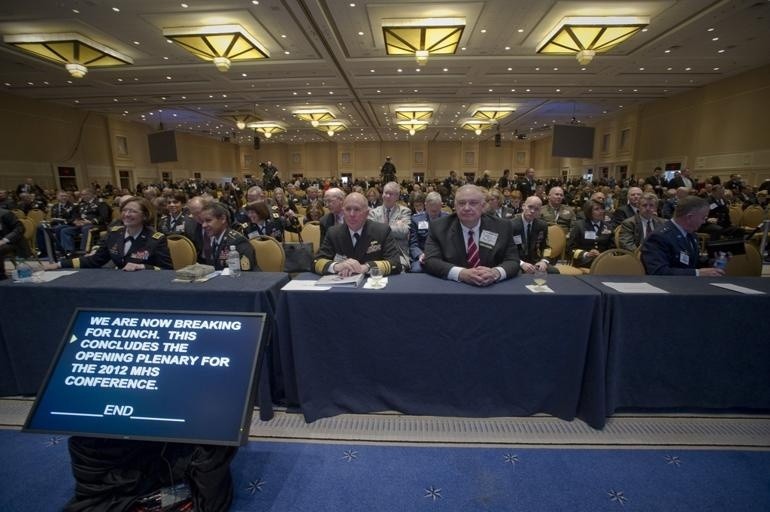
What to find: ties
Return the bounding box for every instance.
[527,224,531,243]
[647,220,652,237]
[385,208,390,225]
[468,231,481,268]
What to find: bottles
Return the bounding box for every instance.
[228,245,241,280]
[715,253,729,270]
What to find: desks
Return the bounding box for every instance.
[272,273,608,430]
[574,273,770,412]
[0,268,289,421]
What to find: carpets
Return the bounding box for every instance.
[0,427,770,512]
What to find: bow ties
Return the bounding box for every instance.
[123,236,134,244]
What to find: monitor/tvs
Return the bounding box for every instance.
[19,306,270,447]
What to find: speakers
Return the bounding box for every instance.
[551,124,594,158]
[147,129,179,164]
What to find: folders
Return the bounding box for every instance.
[314,273,365,288]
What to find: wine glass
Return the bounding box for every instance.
[370,267,384,287]
[534,269,549,292]
[33,264,45,283]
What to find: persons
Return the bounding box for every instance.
[2,162,769,288]
[379,155,397,183]
[260,161,283,186]
